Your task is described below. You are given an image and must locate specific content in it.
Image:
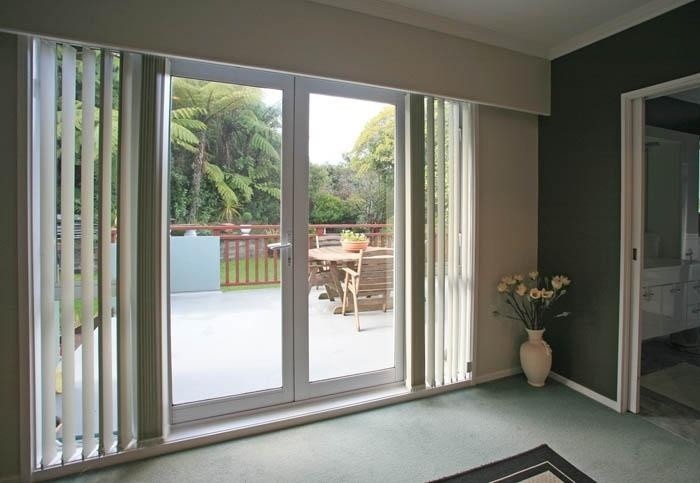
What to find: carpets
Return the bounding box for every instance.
[428,443,597,482]
[637,363,700,448]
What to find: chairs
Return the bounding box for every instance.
[305,233,394,332]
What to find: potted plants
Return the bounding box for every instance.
[341,224,367,253]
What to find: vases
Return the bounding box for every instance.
[519,329,555,388]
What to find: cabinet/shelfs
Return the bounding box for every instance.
[640,264,697,344]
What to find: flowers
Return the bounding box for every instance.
[492,268,570,332]
